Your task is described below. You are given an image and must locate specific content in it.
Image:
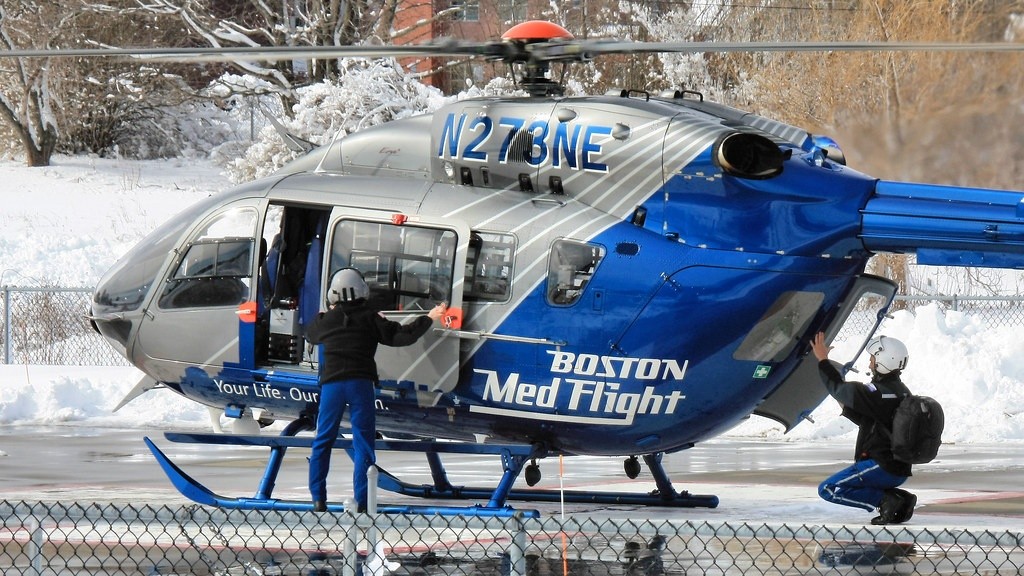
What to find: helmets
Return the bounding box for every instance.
[327,268,369,305]
[866,334,909,375]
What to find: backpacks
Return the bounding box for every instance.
[874,384,944,464]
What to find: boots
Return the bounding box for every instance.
[872,493,902,524]
[890,489,917,523]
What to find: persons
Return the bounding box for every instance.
[809,331,918,526]
[305,268,448,510]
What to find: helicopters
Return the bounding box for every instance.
[0,20,1024,520]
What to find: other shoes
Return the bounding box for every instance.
[313,501,328,512]
[358,506,367,514]
